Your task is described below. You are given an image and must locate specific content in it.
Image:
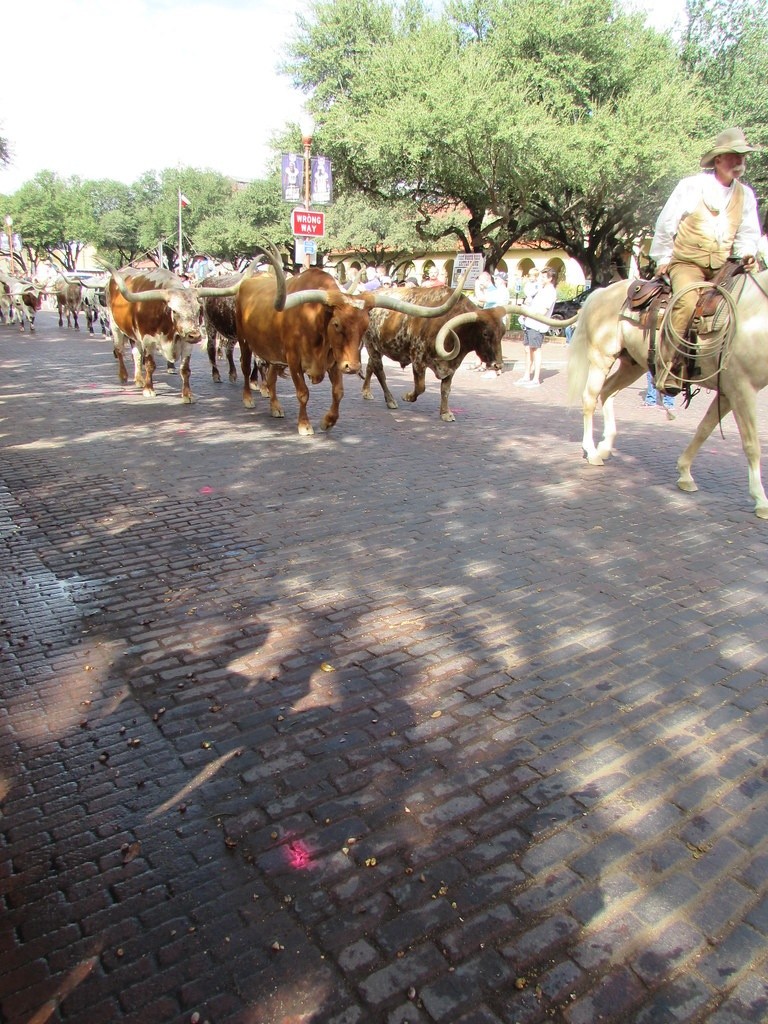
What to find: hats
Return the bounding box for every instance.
[194,252,207,259]
[700,127,758,169]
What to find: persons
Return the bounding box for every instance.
[173,250,215,289]
[421,265,445,287]
[647,127,760,395]
[466,269,510,372]
[514,266,557,388]
[335,265,392,295]
[643,371,674,408]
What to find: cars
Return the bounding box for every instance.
[547,287,600,336]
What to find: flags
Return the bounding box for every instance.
[181,196,192,207]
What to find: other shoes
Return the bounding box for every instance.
[466,365,478,370]
[652,369,680,394]
[473,366,486,372]
[515,377,540,388]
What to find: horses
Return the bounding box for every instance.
[563,266,768,521]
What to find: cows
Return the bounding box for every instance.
[0,267,114,340]
[91,233,580,437]
